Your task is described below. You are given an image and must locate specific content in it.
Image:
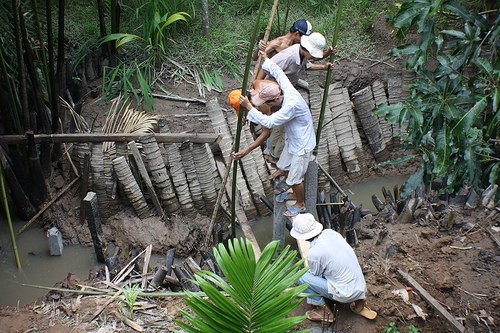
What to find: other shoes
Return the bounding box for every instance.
[273,178,292,195]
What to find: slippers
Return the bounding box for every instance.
[283,205,306,216]
[276,192,297,202]
[306,309,335,322]
[350,302,377,320]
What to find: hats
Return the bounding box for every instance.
[290,213,323,240]
[293,19,312,35]
[301,32,325,58]
[228,90,241,116]
[252,83,281,106]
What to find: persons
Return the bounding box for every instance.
[252,19,337,61]
[228,89,289,195]
[239,50,317,217]
[257,32,335,164]
[290,212,378,322]
